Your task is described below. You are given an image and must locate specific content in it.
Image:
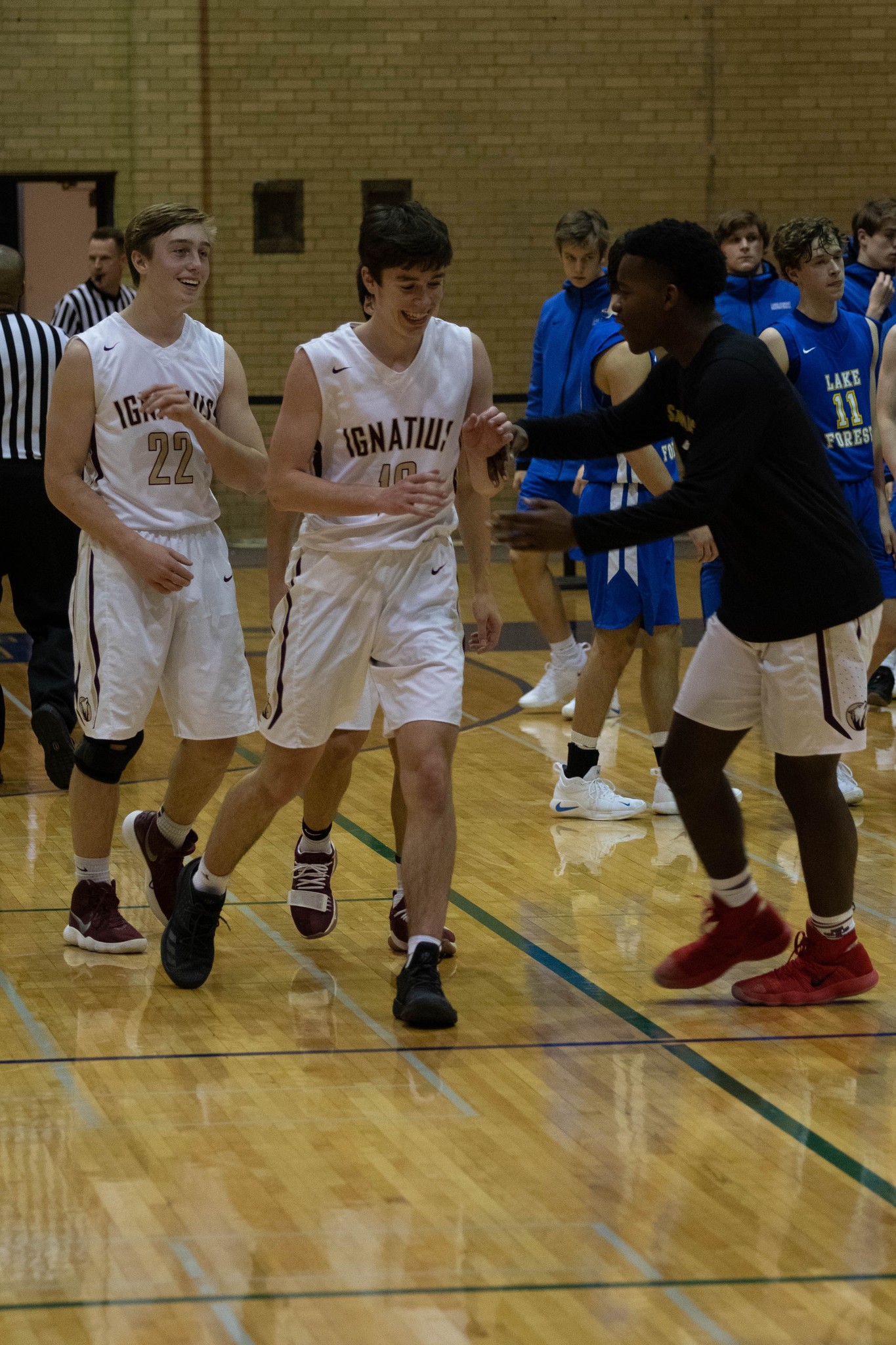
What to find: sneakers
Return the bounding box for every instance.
[652,815,698,872]
[550,819,647,876]
[126,948,184,1057]
[63,949,149,1009]
[388,890,457,956]
[865,704,895,752]
[63,878,148,954]
[731,917,879,1007]
[847,803,864,828]
[394,1024,458,1069]
[561,718,619,752]
[518,642,591,708]
[650,766,681,815]
[835,761,864,805]
[562,687,620,719]
[867,666,895,707]
[122,810,198,927]
[550,762,647,822]
[653,893,792,989]
[393,941,458,1026]
[520,720,563,755]
[288,833,338,939]
[287,964,337,1054]
[160,856,231,990]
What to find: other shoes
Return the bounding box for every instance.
[31,703,76,790]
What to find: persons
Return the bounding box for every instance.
[45,203,268,955]
[1,246,77,792]
[490,220,879,1005]
[51,225,138,337]
[161,204,513,1027]
[509,206,896,823]
